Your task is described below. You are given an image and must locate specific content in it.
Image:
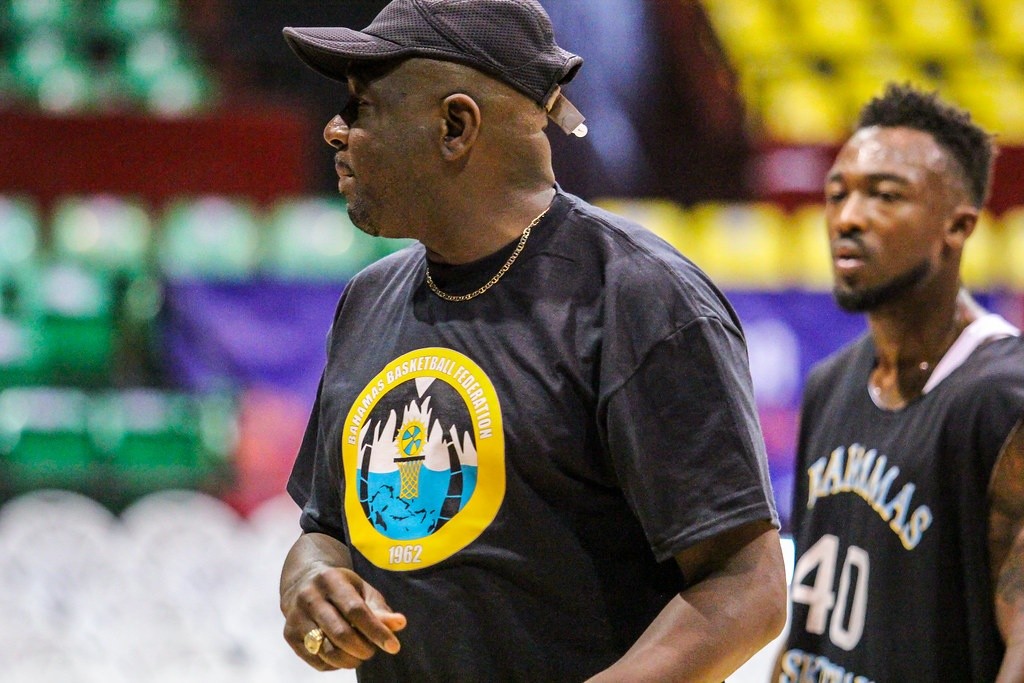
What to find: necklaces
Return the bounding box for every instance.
[874,315,964,380]
[426,208,550,301]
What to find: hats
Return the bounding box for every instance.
[281,0,593,137]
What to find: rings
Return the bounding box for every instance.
[304,629,324,654]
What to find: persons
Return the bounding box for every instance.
[771,84,1024,683]
[279,0,788,683]
